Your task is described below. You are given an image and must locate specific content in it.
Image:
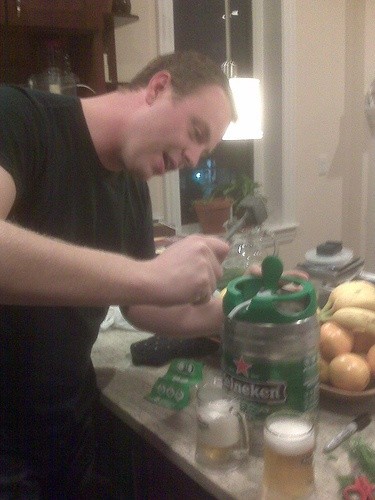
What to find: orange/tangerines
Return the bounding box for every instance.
[316,321,375,393]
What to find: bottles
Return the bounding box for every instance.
[217,256,321,457]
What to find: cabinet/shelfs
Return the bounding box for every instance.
[0,0,139,99]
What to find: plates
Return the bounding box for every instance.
[320,274,375,397]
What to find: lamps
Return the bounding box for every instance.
[221,0,266,140]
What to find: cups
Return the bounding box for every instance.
[263,411,317,500]
[232,233,275,260]
[195,382,251,470]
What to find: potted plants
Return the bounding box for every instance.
[191,172,262,235]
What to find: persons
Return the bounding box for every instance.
[0,52,309,500]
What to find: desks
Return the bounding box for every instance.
[91,304,375,500]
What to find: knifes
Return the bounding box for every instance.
[325,412,372,454]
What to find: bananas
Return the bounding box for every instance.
[316,279,375,335]
